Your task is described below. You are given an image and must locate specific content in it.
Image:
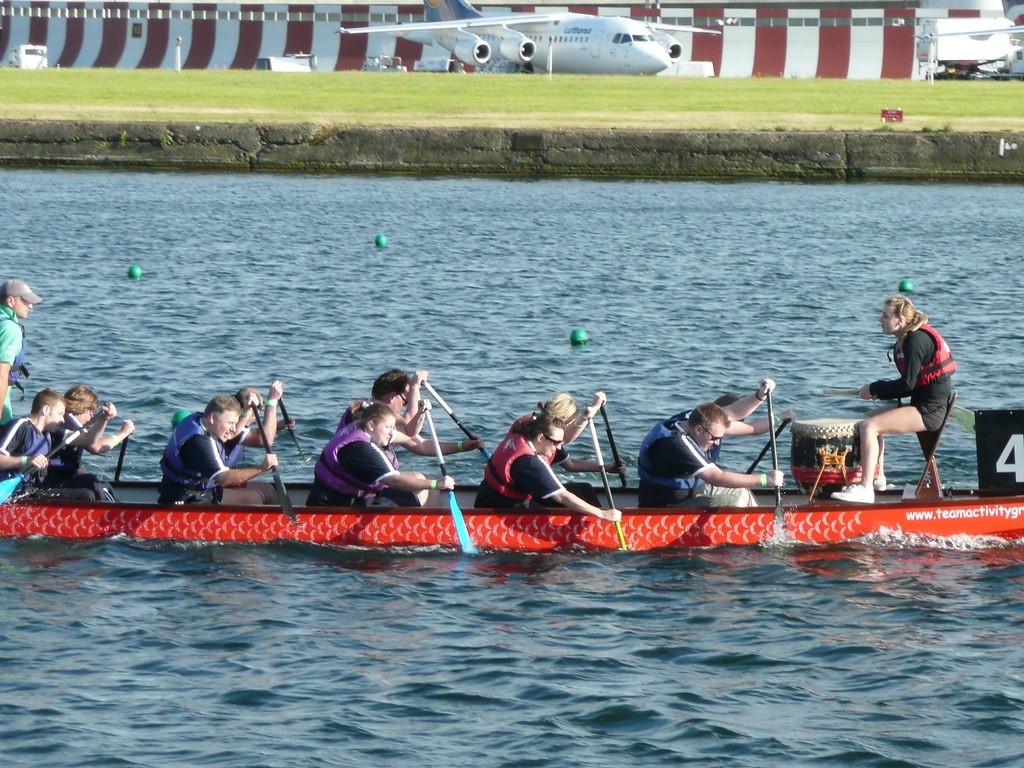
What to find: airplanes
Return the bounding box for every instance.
[335,0,722,77]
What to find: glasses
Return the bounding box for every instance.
[392,389,408,407]
[536,432,561,446]
[696,423,721,441]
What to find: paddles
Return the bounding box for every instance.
[767,390,787,531]
[114,435,131,481]
[599,404,628,489]
[424,409,479,556]
[425,382,492,461]
[250,401,299,528]
[280,398,303,457]
[0,410,105,505]
[745,420,789,474]
[589,416,629,551]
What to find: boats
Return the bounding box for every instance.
[1,481,1024,554]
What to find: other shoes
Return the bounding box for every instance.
[856,472,887,491]
[831,484,875,504]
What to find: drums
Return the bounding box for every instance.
[791,418,880,485]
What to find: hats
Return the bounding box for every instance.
[0,279,43,303]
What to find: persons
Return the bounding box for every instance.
[535,391,628,507]
[222,380,297,503]
[304,401,456,508]
[637,401,785,507]
[0,278,44,438]
[155,393,278,505]
[0,388,117,502]
[472,413,623,522]
[828,294,958,505]
[52,383,134,503]
[670,379,796,468]
[333,367,486,508]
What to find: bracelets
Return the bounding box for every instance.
[265,399,277,406]
[21,456,27,467]
[457,440,465,452]
[762,473,767,486]
[774,414,781,423]
[241,410,246,416]
[431,479,437,490]
[756,391,765,402]
[574,422,583,429]
